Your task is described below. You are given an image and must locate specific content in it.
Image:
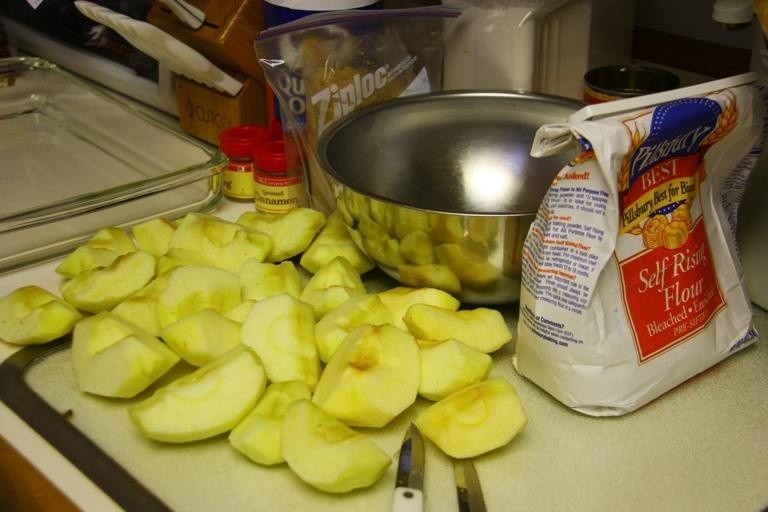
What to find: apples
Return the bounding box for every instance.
[0,206,528,495]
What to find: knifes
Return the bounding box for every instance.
[388,421,425,512]
[454,457,487,512]
[69,0,244,96]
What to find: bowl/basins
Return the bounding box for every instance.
[314,89,590,306]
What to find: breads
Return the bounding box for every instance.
[641,204,692,250]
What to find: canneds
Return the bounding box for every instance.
[582,65,679,105]
